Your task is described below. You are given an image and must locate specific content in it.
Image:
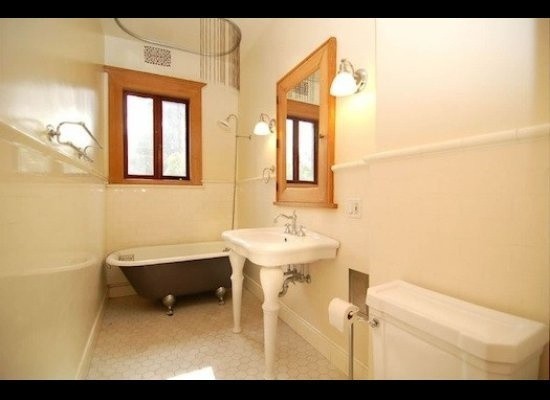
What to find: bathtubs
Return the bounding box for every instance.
[105,241,229,315]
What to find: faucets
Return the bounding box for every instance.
[273,211,297,235]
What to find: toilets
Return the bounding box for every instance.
[365,279,550,380]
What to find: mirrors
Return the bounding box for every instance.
[273,37,337,208]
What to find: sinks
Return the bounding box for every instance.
[222,227,340,267]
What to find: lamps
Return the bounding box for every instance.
[255,114,275,136]
[329,60,367,97]
